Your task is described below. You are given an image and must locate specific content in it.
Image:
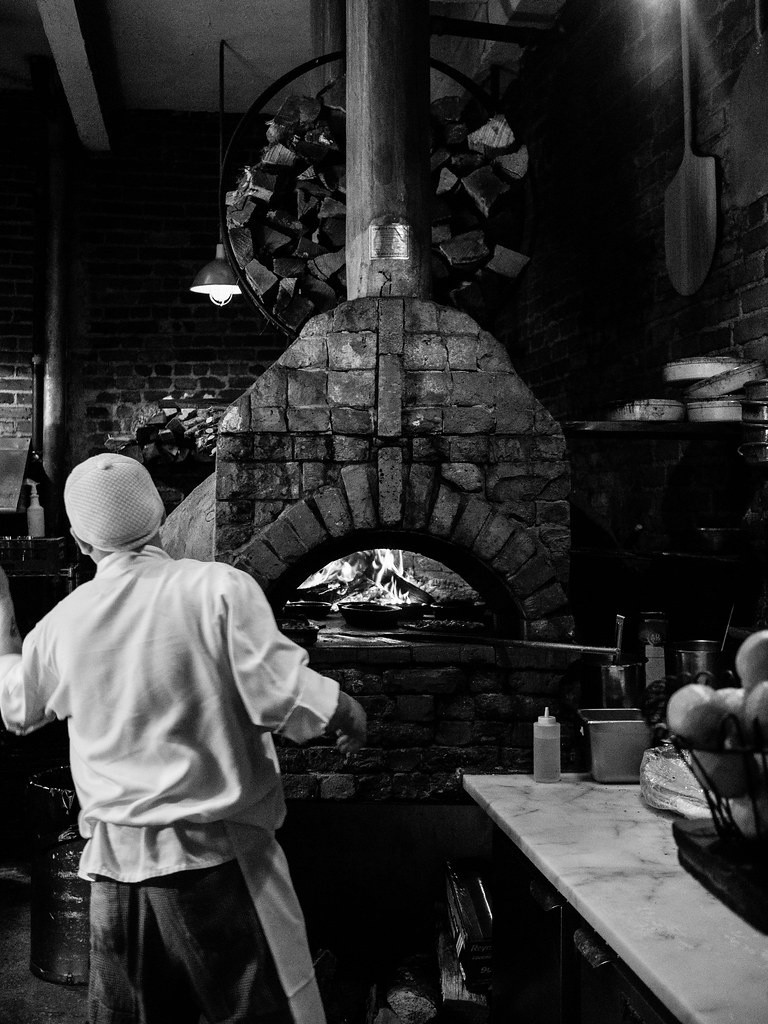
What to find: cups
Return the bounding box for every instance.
[670,639,733,681]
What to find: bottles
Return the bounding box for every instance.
[532,706,560,784]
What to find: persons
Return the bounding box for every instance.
[1,453,366,1024]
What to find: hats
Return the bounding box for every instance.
[65,453,163,547]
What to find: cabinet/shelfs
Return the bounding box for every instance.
[490,801,677,1024]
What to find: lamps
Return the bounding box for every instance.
[188,37,244,306]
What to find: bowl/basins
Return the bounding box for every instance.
[337,602,402,631]
[431,601,475,619]
[398,604,424,620]
[281,627,317,646]
[284,602,332,619]
